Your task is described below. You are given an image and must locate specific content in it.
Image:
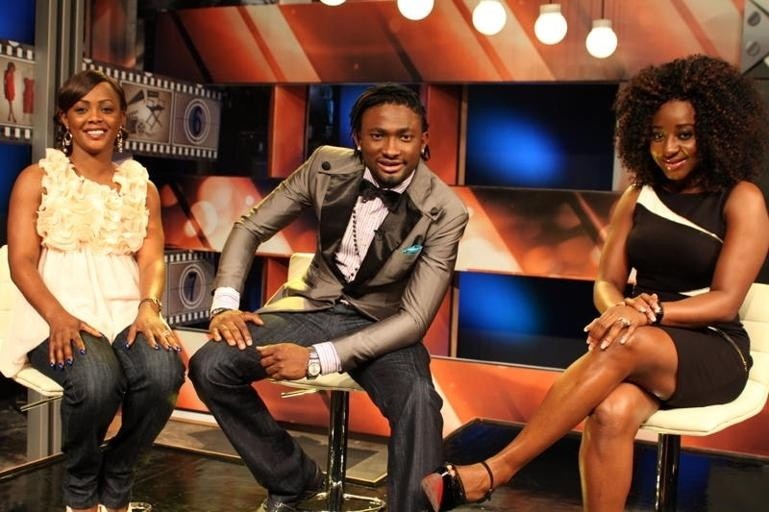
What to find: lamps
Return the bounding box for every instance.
[534,0,568,46]
[585,0,620,59]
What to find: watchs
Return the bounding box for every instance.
[138,297,163,313]
[305,346,322,380]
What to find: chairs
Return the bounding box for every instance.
[1,242,65,412]
[266,253,388,511]
[638,281,768,510]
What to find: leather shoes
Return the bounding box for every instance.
[255,460,328,512]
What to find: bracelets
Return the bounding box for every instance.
[644,298,664,325]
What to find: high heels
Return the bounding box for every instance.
[420,460,495,512]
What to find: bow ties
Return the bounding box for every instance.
[358,179,403,213]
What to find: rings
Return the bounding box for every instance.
[163,329,171,337]
[615,316,630,328]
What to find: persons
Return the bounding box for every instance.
[417,53,769,510]
[1,68,188,512]
[188,81,470,512]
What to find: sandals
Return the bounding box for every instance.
[65,500,153,512]
